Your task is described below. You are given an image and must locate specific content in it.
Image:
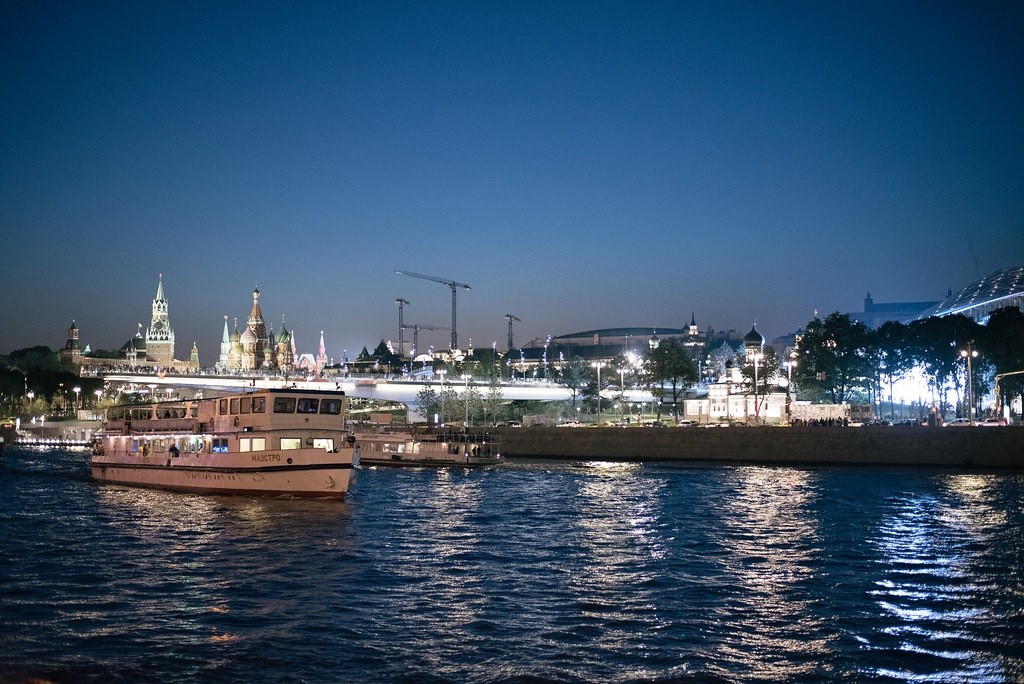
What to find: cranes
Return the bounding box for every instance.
[394,269,472,350]
[396,298,410,371]
[503,312,523,350]
[402,324,451,362]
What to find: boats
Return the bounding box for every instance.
[353,430,506,468]
[89,374,356,497]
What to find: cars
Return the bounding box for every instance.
[644,419,752,428]
[893,417,1006,426]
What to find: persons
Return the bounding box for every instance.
[81,362,434,430]
[92,440,203,457]
[914,415,943,427]
[451,444,490,457]
[790,416,849,427]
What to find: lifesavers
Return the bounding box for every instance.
[234,416,239,427]
[210,418,214,428]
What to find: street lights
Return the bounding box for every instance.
[960,341,979,425]
[460,374,473,428]
[616,369,628,423]
[73,386,81,421]
[27,391,34,411]
[592,362,605,426]
[749,352,764,427]
[94,390,102,421]
[146,385,159,396]
[782,360,798,426]
[166,388,173,401]
[435,369,448,428]
